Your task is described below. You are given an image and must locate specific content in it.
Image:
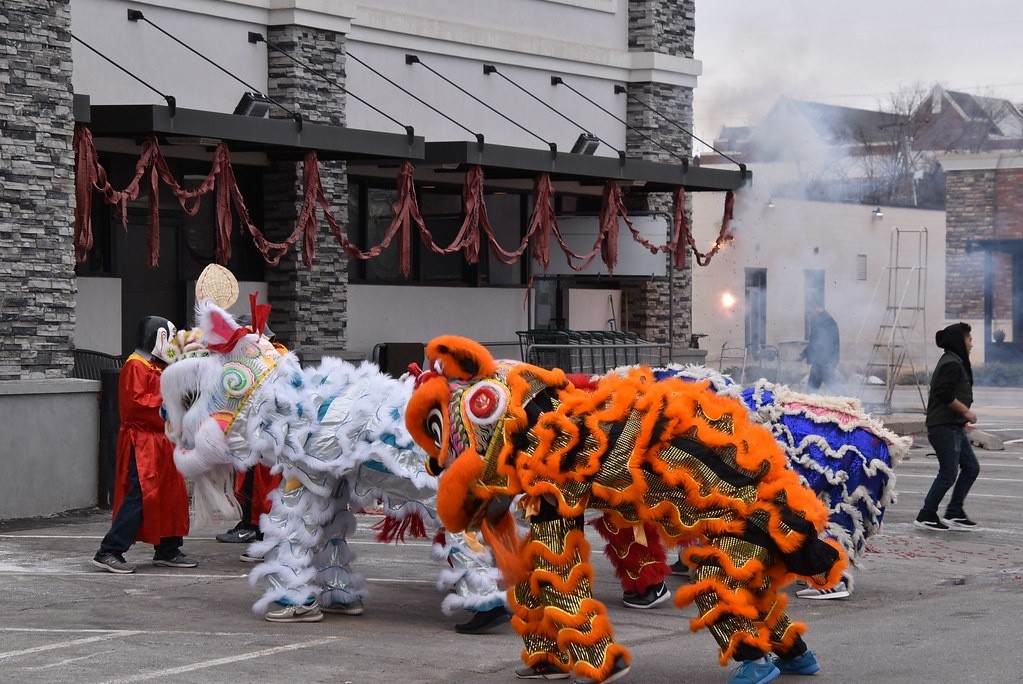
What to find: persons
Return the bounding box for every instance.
[912,323,981,532]
[796,297,843,396]
[216,308,288,544]
[92,316,198,573]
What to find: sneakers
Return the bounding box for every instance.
[622,580,672,609]
[216,526,256,543]
[795,581,849,599]
[239,553,264,562]
[153,551,198,567]
[669,560,690,576]
[724,650,820,684]
[265,599,363,623]
[454,606,510,632]
[515,655,630,684]
[92,550,137,573]
[914,509,978,531]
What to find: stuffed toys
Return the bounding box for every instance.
[404,335,851,684]
[158,302,522,637]
[649,363,913,600]
